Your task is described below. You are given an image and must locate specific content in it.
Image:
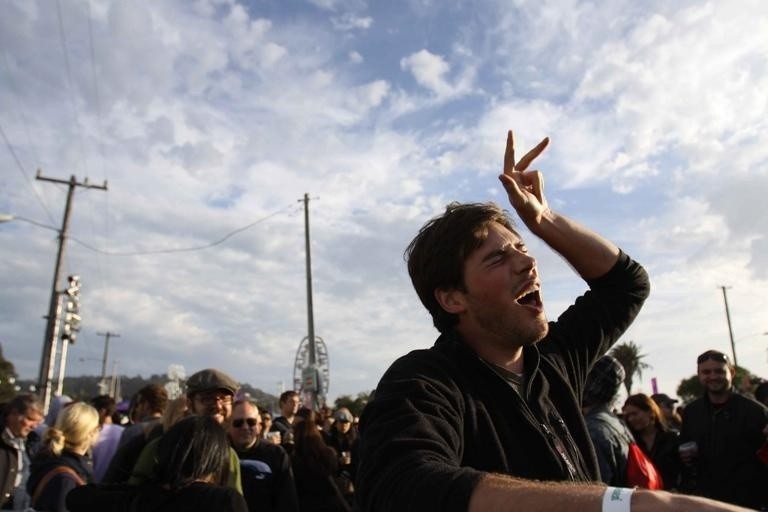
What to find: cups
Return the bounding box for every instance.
[342,450,352,466]
[679,442,700,471]
[267,432,280,444]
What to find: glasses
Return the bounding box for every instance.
[190,396,234,407]
[697,353,728,365]
[232,418,259,428]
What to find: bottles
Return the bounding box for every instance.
[282,431,295,455]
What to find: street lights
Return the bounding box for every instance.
[0,213,66,418]
[54,272,84,399]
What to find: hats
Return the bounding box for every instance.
[651,394,679,407]
[586,355,626,402]
[334,410,354,424]
[185,369,241,398]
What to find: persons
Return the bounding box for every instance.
[582,350,767,512]
[0,370,375,512]
[360,129,753,511]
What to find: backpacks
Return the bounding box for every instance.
[589,416,665,490]
